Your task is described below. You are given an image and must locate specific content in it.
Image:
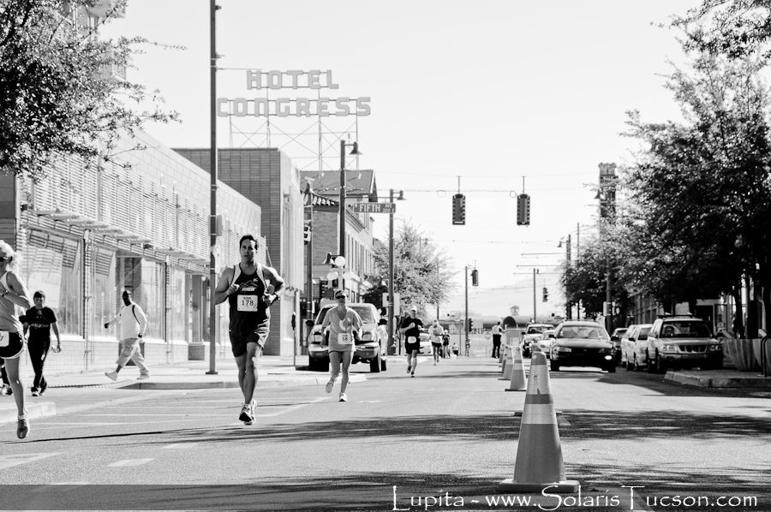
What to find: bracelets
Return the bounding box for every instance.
[1,292,9,298]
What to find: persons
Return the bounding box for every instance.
[213,234,285,425]
[429,319,459,364]
[104,290,150,380]
[22,292,62,396]
[399,306,425,377]
[0,240,31,438]
[492,322,504,358]
[321,291,362,402]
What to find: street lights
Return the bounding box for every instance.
[322,138,363,293]
[387,188,406,354]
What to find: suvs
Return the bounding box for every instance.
[646,313,725,375]
[522,324,554,353]
[306,301,389,374]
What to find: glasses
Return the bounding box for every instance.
[336,296,345,299]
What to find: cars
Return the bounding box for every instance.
[537,329,557,359]
[610,323,653,371]
[548,320,618,376]
[419,333,434,355]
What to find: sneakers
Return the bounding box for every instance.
[31,387,40,397]
[339,392,347,402]
[105,371,118,382]
[138,373,151,380]
[325,379,334,393]
[239,402,253,422]
[434,355,450,366]
[407,365,412,373]
[16,410,31,439]
[244,398,257,425]
[411,372,415,377]
[40,381,49,396]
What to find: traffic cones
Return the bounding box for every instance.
[496,351,582,491]
[505,346,532,392]
[499,344,515,380]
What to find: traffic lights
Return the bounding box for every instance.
[541,287,549,302]
[468,317,475,332]
